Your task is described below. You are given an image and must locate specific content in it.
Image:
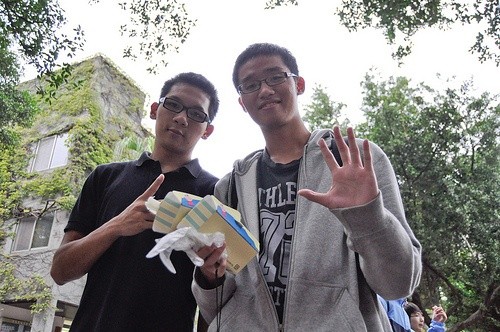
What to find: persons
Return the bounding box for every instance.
[191,41,424,332]
[376,292,448,332]
[402,302,425,332]
[49,72,224,331]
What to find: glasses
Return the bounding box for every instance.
[159,97,211,125]
[237,73,296,95]
